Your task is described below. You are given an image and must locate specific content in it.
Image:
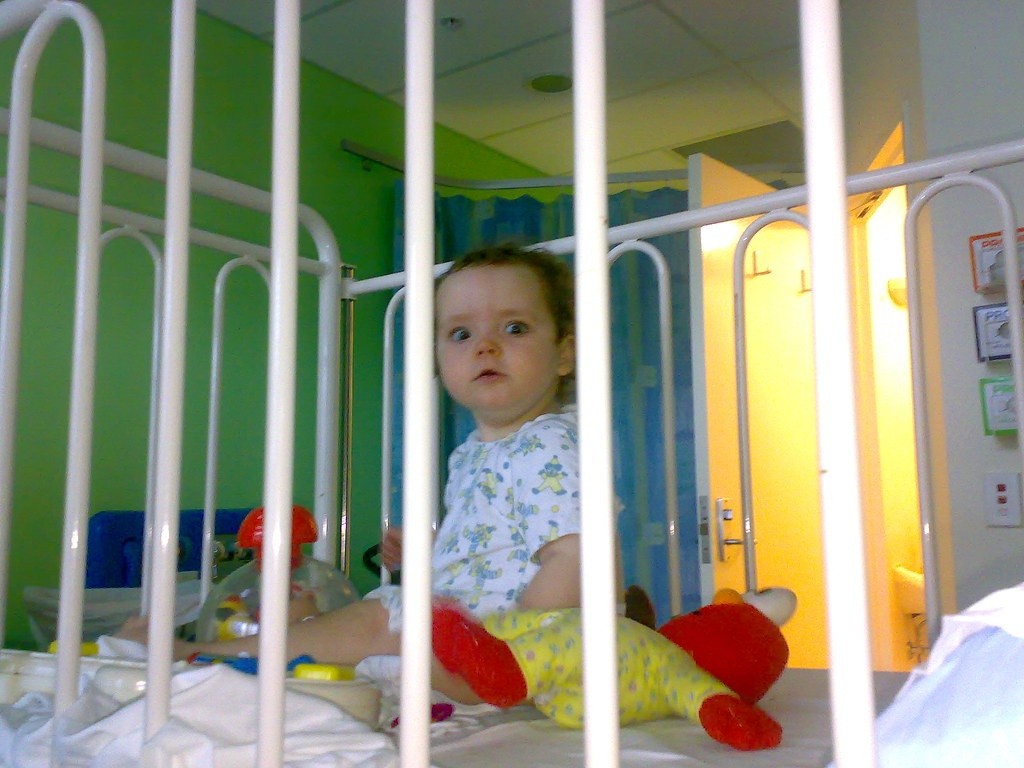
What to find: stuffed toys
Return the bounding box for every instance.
[415,585,800,752]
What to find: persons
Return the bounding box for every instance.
[116,244,626,710]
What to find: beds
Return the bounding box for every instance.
[0,0,1024,768]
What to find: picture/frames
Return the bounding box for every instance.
[979,376,1017,436]
[972,302,1024,362]
[969,227,1024,293]
